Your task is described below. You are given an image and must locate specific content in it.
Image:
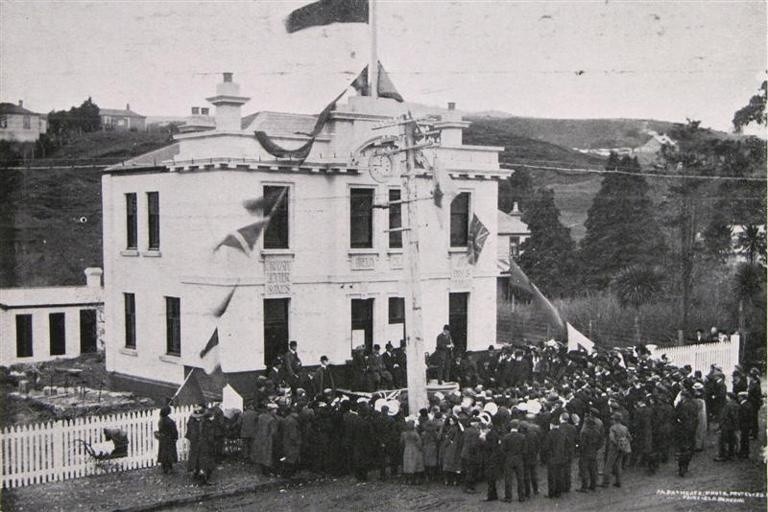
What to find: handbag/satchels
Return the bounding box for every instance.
[618,437,634,454]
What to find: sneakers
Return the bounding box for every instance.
[264,465,623,505]
[711,450,751,464]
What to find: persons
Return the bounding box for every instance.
[152,319,762,503]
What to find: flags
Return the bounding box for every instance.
[207,281,239,317]
[243,188,282,216]
[210,217,269,256]
[283,0,371,35]
[507,262,529,294]
[353,62,403,102]
[297,88,347,145]
[430,157,458,232]
[465,213,490,268]
[529,282,566,326]
[255,129,313,163]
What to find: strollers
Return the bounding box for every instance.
[73,427,130,474]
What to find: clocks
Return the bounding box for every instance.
[367,151,395,183]
